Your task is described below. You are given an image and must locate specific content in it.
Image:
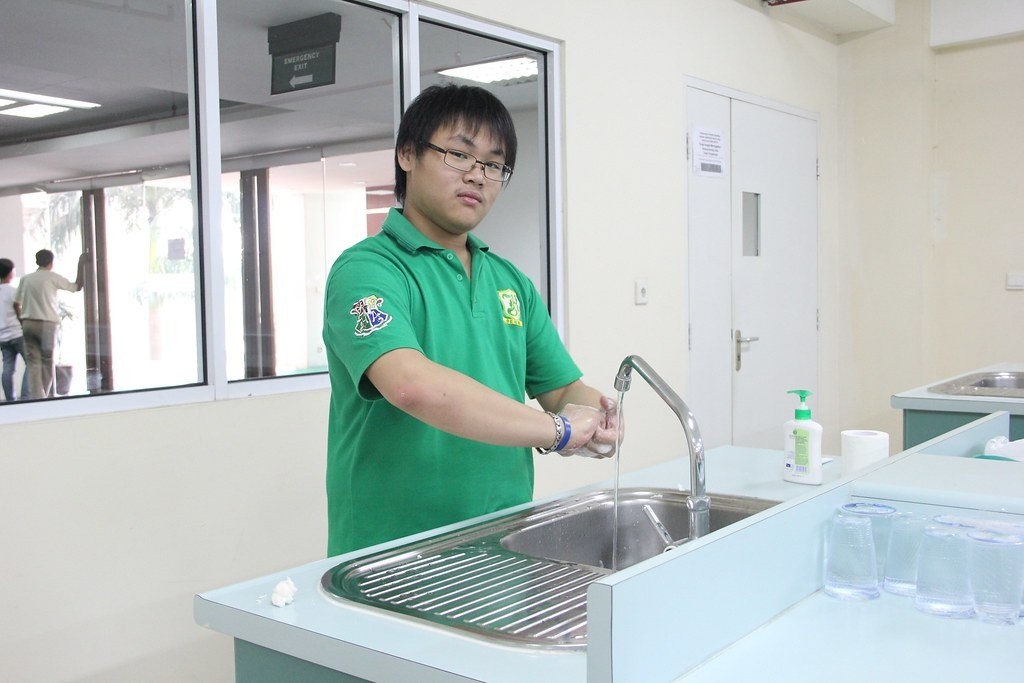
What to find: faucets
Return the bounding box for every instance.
[613,354,711,540]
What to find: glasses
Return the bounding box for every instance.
[420,141,513,182]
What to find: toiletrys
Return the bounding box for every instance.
[783,389,823,485]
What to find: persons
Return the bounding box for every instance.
[0,258,31,400]
[321,83,625,559]
[13,249,88,399]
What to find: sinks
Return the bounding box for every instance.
[495,487,784,574]
[926,371,1024,398]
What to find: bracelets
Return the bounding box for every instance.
[554,416,571,452]
[535,410,562,455]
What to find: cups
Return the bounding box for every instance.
[821,503,1024,623]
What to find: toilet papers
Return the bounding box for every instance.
[840,430,889,477]
